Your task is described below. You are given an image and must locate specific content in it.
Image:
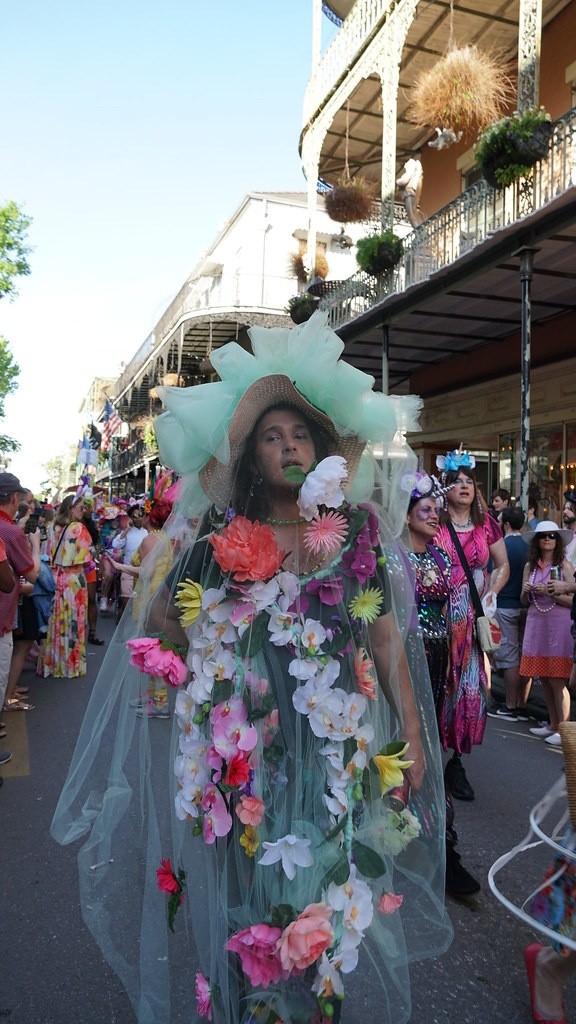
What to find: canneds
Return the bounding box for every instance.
[549,566,561,596]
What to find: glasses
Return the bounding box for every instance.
[131,513,144,518]
[72,506,86,511]
[536,533,558,540]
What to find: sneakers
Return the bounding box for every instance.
[516,708,529,722]
[134,703,170,718]
[444,758,474,799]
[130,691,153,707]
[434,850,480,898]
[487,705,518,721]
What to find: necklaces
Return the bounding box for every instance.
[414,556,435,587]
[530,565,561,612]
[279,551,327,575]
[451,517,472,528]
[265,515,306,527]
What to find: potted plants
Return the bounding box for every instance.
[355,229,403,274]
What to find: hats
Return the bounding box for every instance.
[0,473,28,495]
[521,521,574,547]
[100,507,127,520]
[199,374,366,518]
[564,488,576,502]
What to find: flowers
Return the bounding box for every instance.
[472,103,552,188]
[283,291,314,314]
[126,456,422,1024]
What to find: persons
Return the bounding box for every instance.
[433,453,510,798]
[56,318,450,1024]
[0,471,199,776]
[477,486,576,740]
[389,471,479,899]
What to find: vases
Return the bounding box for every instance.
[291,299,320,324]
[483,120,553,191]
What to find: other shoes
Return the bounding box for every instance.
[529,727,553,736]
[0,687,35,764]
[88,634,105,645]
[545,732,562,745]
[100,597,109,610]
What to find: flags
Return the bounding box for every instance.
[74,424,101,466]
[102,399,122,451]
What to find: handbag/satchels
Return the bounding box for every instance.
[477,616,501,653]
